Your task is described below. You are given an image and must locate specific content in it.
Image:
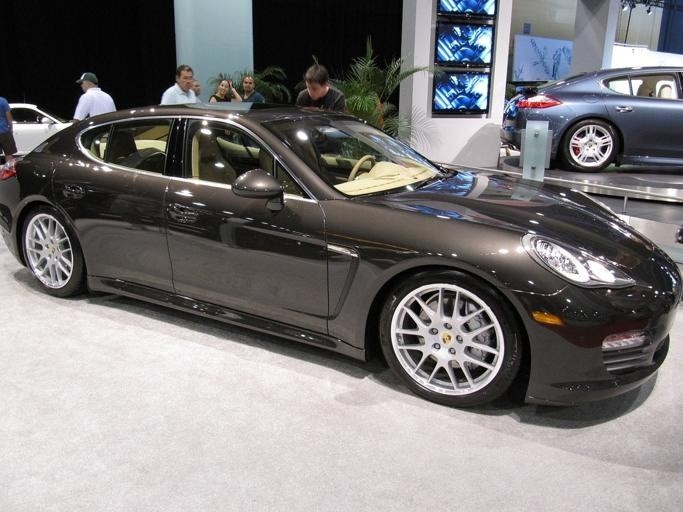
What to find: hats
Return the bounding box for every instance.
[76,72,98,84]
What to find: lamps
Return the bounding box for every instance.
[620,1,653,16]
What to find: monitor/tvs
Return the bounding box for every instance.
[433,20,496,68]
[436,0,500,20]
[432,67,493,118]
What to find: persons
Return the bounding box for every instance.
[209,80,242,104]
[238,76,266,105]
[293,64,345,114]
[0,94,17,162]
[161,64,196,105]
[72,72,117,126]
[192,80,202,104]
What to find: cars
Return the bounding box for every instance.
[2,103,77,159]
[2,103,683,410]
[501,65,683,172]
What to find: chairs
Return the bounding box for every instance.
[259,148,301,196]
[113,130,154,172]
[189,126,238,189]
